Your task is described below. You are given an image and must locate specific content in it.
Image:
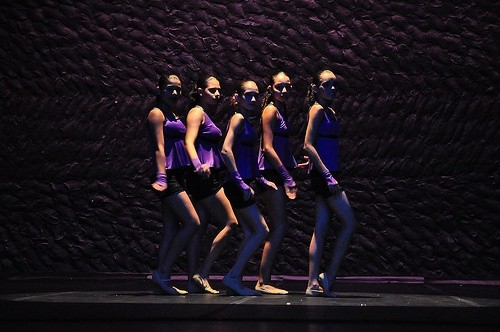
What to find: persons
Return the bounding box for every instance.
[254,70,299,295]
[218,77,271,295]
[303,70,354,296]
[183,76,239,294]
[145,73,201,297]
